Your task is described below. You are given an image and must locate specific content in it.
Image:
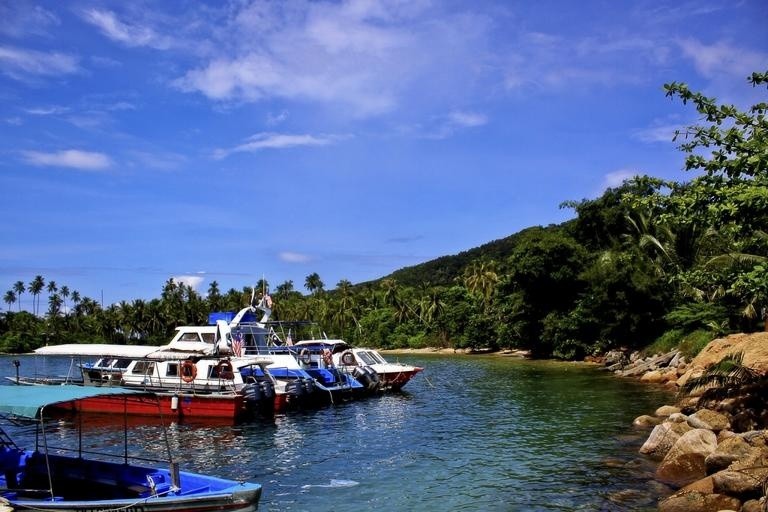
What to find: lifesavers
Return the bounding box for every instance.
[300,348,311,365]
[342,352,355,366]
[177,361,197,382]
[216,360,233,379]
